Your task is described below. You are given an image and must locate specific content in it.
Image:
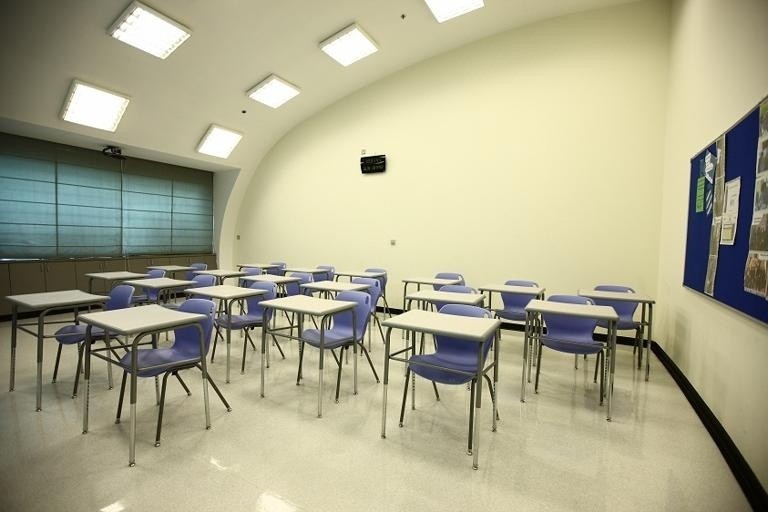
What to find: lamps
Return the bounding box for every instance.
[194,123,244,160]
[426,1,485,23]
[317,23,382,72]
[57,80,133,133]
[105,1,192,59]
[245,73,304,114]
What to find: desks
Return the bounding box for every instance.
[578,288,656,384]
[7,289,114,411]
